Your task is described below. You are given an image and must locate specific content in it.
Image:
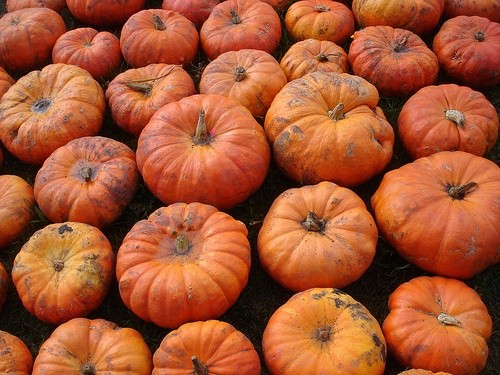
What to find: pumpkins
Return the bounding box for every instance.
[0,0,500,375]
